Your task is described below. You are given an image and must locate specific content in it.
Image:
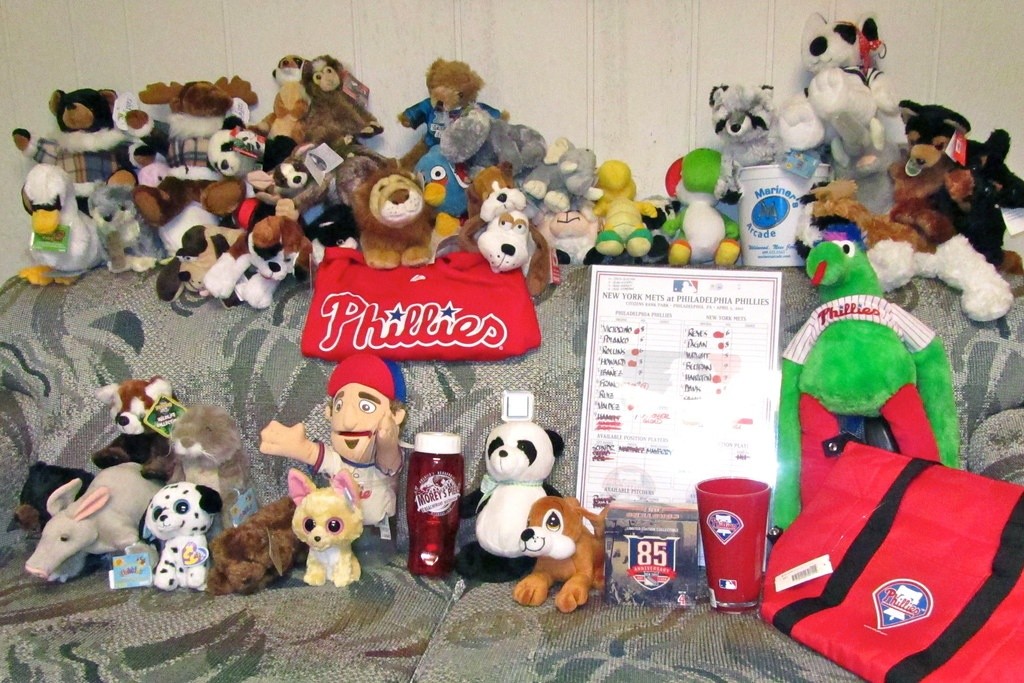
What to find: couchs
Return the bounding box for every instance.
[0,241,1024,682]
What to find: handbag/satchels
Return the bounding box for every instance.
[760,443,1023,683]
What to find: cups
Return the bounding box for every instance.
[693,476,771,615]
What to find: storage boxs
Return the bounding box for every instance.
[734,164,827,269]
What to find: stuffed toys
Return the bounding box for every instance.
[24,461,164,584]
[770,214,961,544]
[207,496,302,595]
[139,482,223,592]
[259,353,411,566]
[455,421,566,583]
[511,495,613,614]
[167,406,260,543]
[286,467,365,587]
[90,375,172,469]
[10,13,1022,324]
[7,461,96,532]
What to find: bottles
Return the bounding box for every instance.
[405,431,463,578]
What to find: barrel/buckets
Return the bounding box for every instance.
[735,163,831,269]
